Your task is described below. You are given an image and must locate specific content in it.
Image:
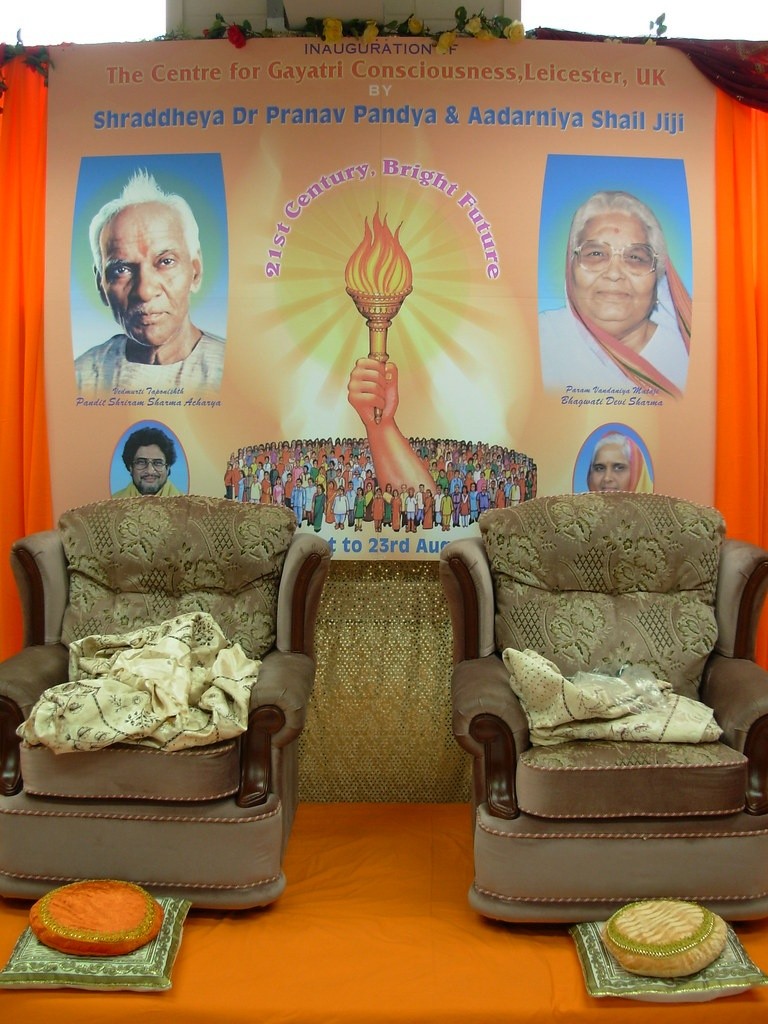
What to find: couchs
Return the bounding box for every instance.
[431,489,768,922]
[0,492,333,911]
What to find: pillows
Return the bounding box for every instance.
[566,900,768,1004]
[0,881,192,992]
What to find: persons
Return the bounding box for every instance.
[71,167,225,394]
[587,431,653,493]
[538,192,689,391]
[112,426,185,499]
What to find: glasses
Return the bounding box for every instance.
[131,459,168,472]
[574,240,658,276]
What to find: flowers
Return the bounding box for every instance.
[1,4,666,93]
